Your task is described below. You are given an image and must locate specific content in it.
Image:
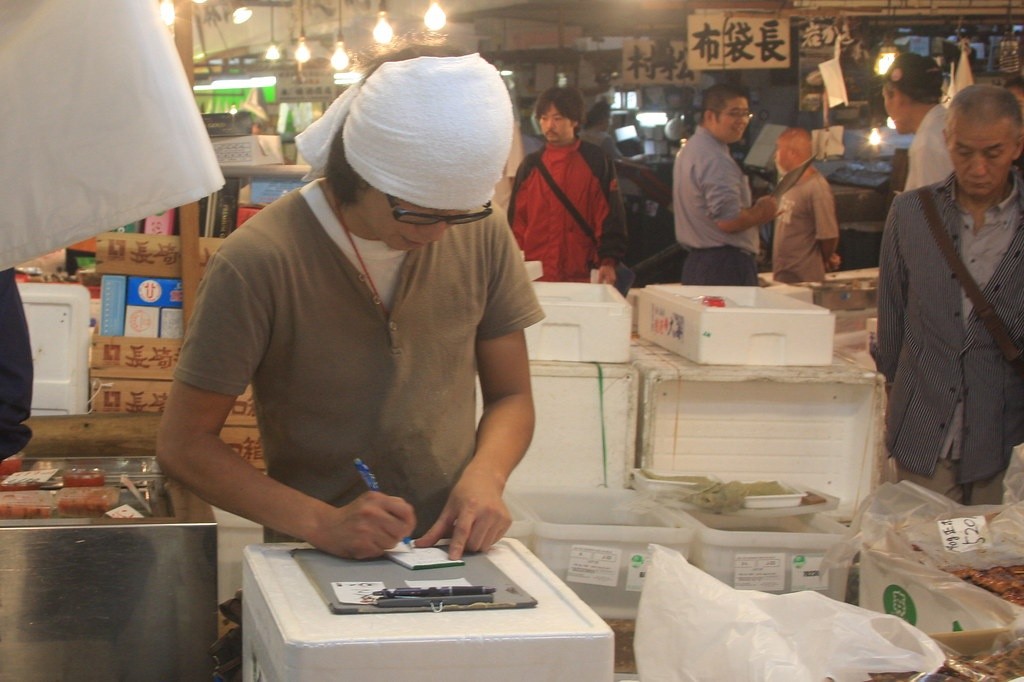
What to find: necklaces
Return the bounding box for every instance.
[336,202,389,320]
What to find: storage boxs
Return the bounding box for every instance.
[202,112,284,167]
[85,232,1024,682]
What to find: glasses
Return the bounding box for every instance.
[387,191,494,225]
[711,108,754,121]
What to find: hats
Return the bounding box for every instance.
[887,53,943,104]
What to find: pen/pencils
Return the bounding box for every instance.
[373,585,496,607]
[352,458,417,553]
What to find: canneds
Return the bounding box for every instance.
[701,295,725,307]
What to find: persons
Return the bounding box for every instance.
[882,52,960,191]
[773,126,841,285]
[157,38,547,562]
[578,101,622,160]
[874,85,1023,506]
[508,87,628,285]
[672,83,784,287]
